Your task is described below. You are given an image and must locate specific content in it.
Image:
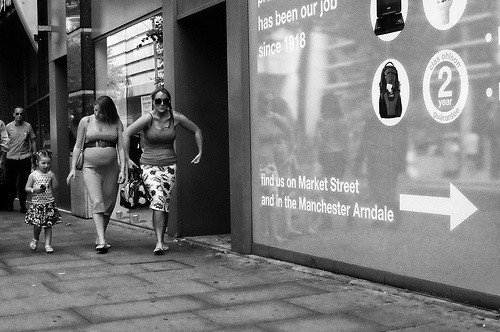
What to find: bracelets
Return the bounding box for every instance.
[31,188,34,193]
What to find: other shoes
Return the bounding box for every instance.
[30,238,37,250]
[95,239,110,247]
[45,245,53,252]
[96,244,108,251]
[156,242,169,251]
[9,207,14,211]
[153,242,164,253]
[20,208,27,214]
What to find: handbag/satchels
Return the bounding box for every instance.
[75,153,84,170]
[120,171,152,210]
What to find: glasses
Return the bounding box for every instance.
[15,112,23,115]
[153,98,170,104]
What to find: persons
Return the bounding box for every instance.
[0,107,63,253]
[66,96,126,252]
[123,88,203,254]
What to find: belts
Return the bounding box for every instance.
[86,140,117,148]
